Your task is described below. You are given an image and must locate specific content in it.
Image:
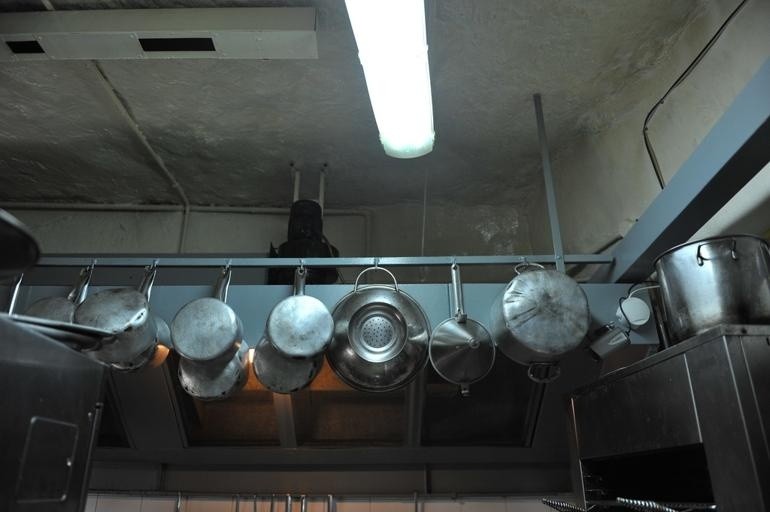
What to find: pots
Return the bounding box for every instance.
[175,339,251,400]
[250,334,326,396]
[324,261,431,396]
[26,262,159,375]
[168,266,244,365]
[263,265,336,362]
[489,262,590,385]
[652,235,770,344]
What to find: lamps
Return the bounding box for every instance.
[345,0,436,159]
